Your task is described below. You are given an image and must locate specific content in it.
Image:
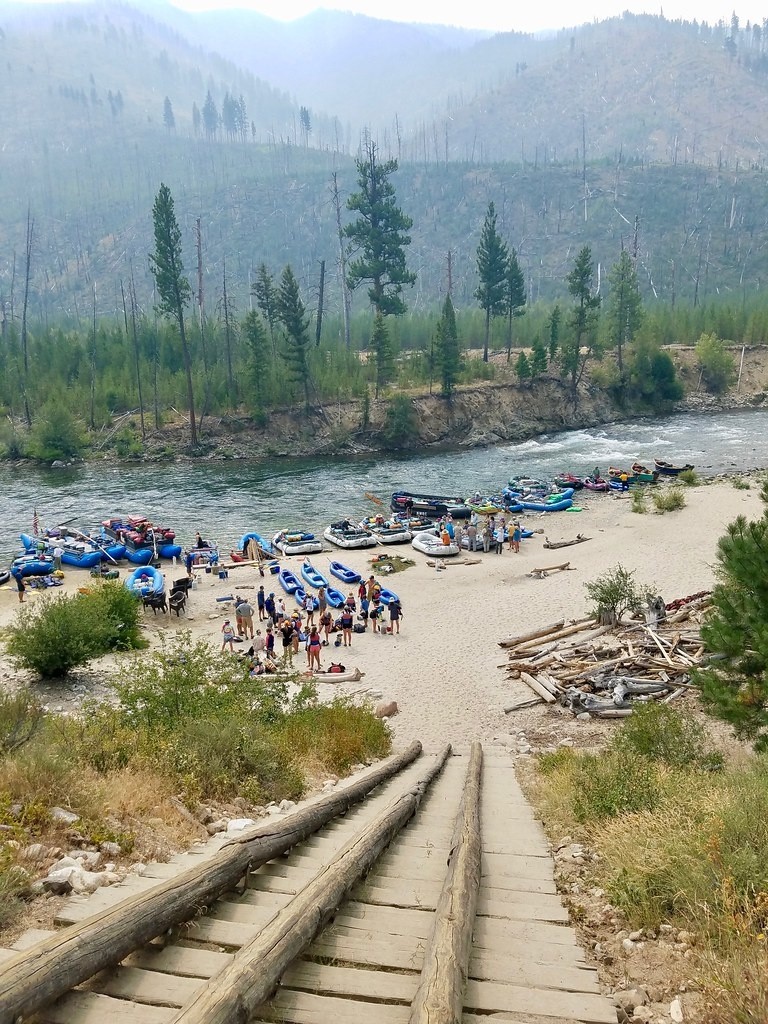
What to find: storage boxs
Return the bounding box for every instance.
[205,563,228,579]
[270,564,282,574]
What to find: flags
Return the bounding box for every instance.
[33,506,39,535]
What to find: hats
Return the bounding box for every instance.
[359,575,380,590]
[446,511,451,515]
[184,550,191,554]
[499,516,505,521]
[223,618,231,623]
[497,526,504,532]
[376,513,382,518]
[325,611,331,618]
[508,516,519,524]
[389,597,395,602]
[344,604,349,609]
[348,591,354,598]
[269,592,275,597]
[469,521,475,525]
[345,517,352,521]
[441,528,449,534]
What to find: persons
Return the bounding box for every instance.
[221,575,402,675]
[341,517,354,535]
[504,493,511,512]
[42,537,50,553]
[139,522,149,534]
[207,549,217,566]
[474,492,482,502]
[185,552,197,579]
[404,497,413,518]
[280,532,285,541]
[15,569,26,603]
[375,514,384,528]
[141,574,150,583]
[53,545,65,571]
[591,465,600,484]
[433,511,521,554]
[619,472,629,492]
[195,532,203,549]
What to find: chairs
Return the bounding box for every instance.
[140,576,195,617]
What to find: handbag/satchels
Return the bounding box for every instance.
[232,646,282,675]
[354,623,365,633]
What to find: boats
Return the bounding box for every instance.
[238,532,273,560]
[300,563,329,589]
[323,522,377,550]
[295,589,318,610]
[654,458,695,475]
[0,572,10,585]
[410,532,460,557]
[464,497,499,515]
[9,514,182,576]
[492,527,534,538]
[609,481,628,491]
[631,462,654,481]
[125,565,163,600]
[488,495,525,512]
[325,588,346,608]
[502,474,575,499]
[460,535,497,551]
[360,516,411,545]
[553,476,583,490]
[389,512,438,537]
[278,569,304,594]
[270,528,323,555]
[391,491,473,519]
[511,496,573,512]
[583,476,610,491]
[378,589,399,606]
[185,539,219,564]
[607,466,636,483]
[330,561,362,583]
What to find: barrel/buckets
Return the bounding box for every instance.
[218,569,228,578]
[197,575,202,583]
[206,568,211,573]
[270,565,280,573]
[192,580,197,590]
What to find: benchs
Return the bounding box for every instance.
[422,539,450,546]
[134,581,153,587]
[135,575,153,582]
